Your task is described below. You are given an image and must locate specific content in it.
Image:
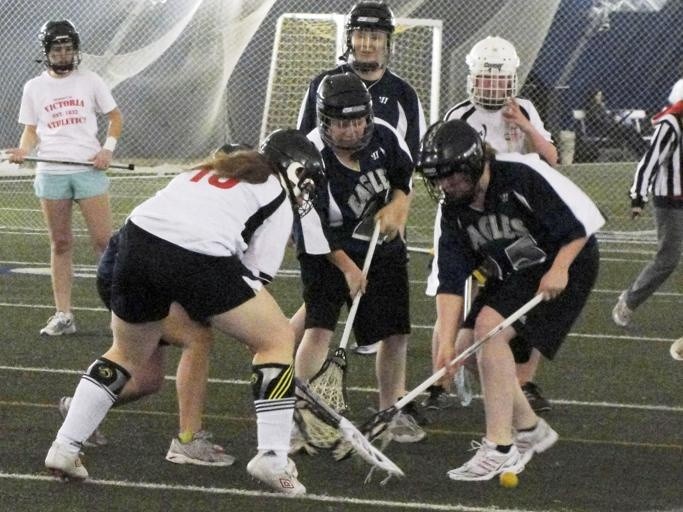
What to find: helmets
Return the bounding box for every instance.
[340,2,396,70]
[260,127,326,221]
[38,18,81,77]
[316,70,375,153]
[465,36,520,113]
[416,121,483,208]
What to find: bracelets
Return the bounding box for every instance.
[103,136,117,152]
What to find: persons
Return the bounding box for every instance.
[420,35,558,412]
[59,145,253,466]
[413,120,604,482]
[5,19,122,338]
[287,3,421,351]
[291,74,426,458]
[589,87,649,160]
[611,98,683,364]
[43,131,339,495]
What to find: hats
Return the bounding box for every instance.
[667,79,682,104]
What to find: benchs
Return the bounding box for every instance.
[572,108,652,148]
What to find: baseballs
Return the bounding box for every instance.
[499,470,519,490]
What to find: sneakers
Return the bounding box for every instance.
[246,451,306,495]
[59,397,109,449]
[364,361,560,443]
[45,444,89,480]
[289,422,308,453]
[512,418,560,468]
[446,436,526,484]
[167,430,235,467]
[669,338,682,361]
[40,311,76,336]
[350,340,383,354]
[611,289,633,327]
